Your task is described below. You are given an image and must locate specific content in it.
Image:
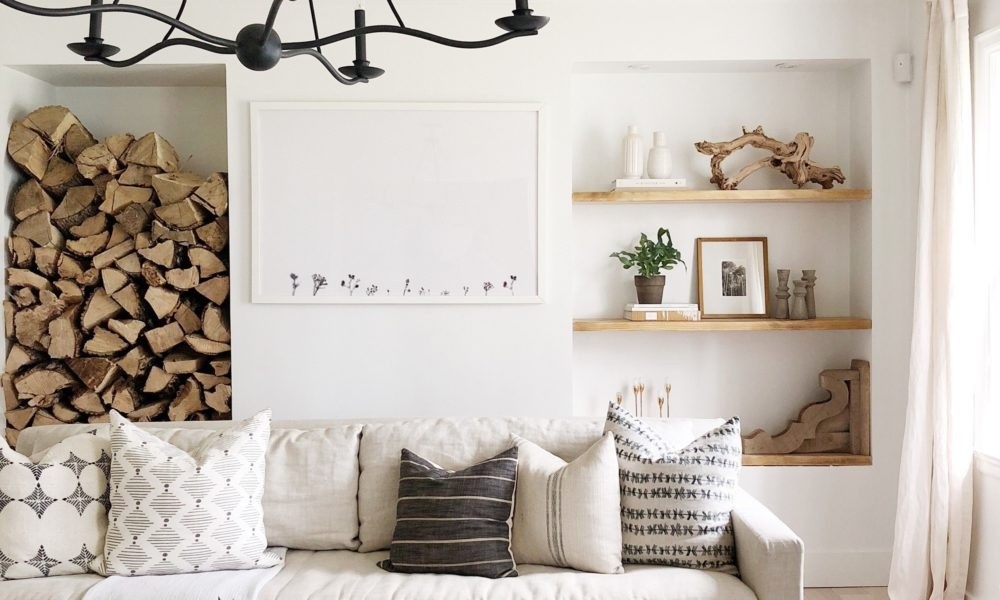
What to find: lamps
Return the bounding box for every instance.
[1,0,553,84]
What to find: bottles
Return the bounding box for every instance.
[622,125,644,179]
[647,131,673,179]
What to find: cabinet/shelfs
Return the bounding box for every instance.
[567,188,872,470]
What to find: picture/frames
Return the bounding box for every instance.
[698,236,770,320]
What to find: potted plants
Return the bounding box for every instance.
[609,226,689,305]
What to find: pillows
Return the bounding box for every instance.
[506,435,629,574]
[150,425,364,552]
[378,449,517,578]
[358,423,599,555]
[0,429,108,578]
[598,403,740,576]
[101,412,283,577]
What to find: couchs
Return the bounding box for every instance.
[1,416,803,600]
